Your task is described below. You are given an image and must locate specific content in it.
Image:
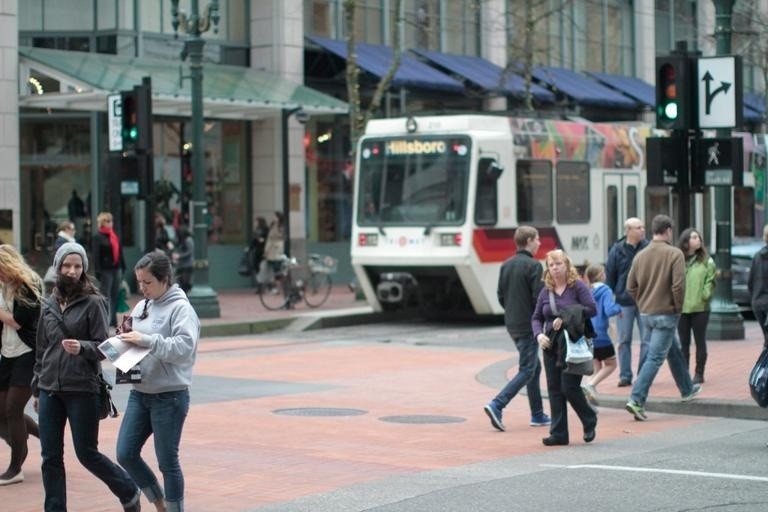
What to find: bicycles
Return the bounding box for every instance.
[253,252,337,311]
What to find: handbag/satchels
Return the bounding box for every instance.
[116,362,142,384]
[97,375,119,419]
[116,286,130,312]
[564,329,595,375]
[238,248,251,277]
[256,259,276,285]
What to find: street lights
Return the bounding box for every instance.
[168,1,223,325]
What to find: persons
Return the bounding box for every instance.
[167,226,196,292]
[580,262,626,414]
[29,240,143,511]
[90,210,128,327]
[264,210,304,310]
[153,215,170,248]
[483,224,553,434]
[54,220,78,253]
[747,225,768,349]
[674,227,719,384]
[530,248,600,447]
[242,215,269,295]
[605,217,651,388]
[625,213,704,422]
[0,242,47,485]
[114,247,203,511]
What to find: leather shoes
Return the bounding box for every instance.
[0,469,23,485]
[125,489,142,512]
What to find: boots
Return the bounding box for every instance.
[691,354,707,383]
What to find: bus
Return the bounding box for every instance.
[343,102,767,328]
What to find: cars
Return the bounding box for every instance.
[703,238,764,318]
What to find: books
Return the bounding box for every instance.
[98,332,152,373]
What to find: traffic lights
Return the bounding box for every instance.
[652,50,699,134]
[120,90,138,159]
[690,137,744,192]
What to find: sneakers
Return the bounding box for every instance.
[542,435,569,445]
[581,383,601,407]
[618,380,631,387]
[682,383,701,402]
[531,414,550,425]
[485,400,504,432]
[583,430,595,442]
[626,399,647,420]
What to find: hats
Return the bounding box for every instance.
[53,242,89,276]
